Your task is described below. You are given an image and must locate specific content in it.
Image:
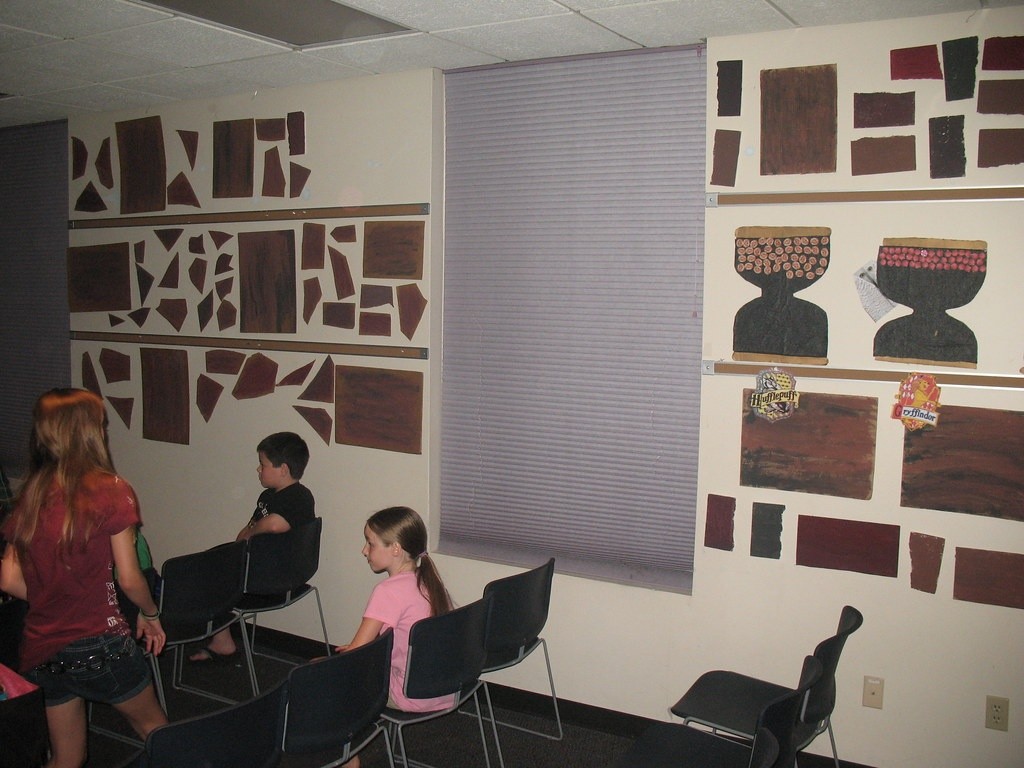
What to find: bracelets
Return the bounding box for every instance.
[140,608,160,619]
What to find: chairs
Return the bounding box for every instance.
[671,606,863,768]
[265,626,394,768]
[177,517,332,704]
[616,655,823,768]
[139,679,288,768]
[458,557,564,768]
[88,538,258,747]
[380,590,496,768]
[45,566,165,768]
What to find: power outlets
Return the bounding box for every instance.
[984,696,1009,731]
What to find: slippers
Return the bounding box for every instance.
[183,643,242,666]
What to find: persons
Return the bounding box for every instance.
[191,432,315,661]
[112,527,151,580]
[336,507,457,768]
[0,388,170,768]
